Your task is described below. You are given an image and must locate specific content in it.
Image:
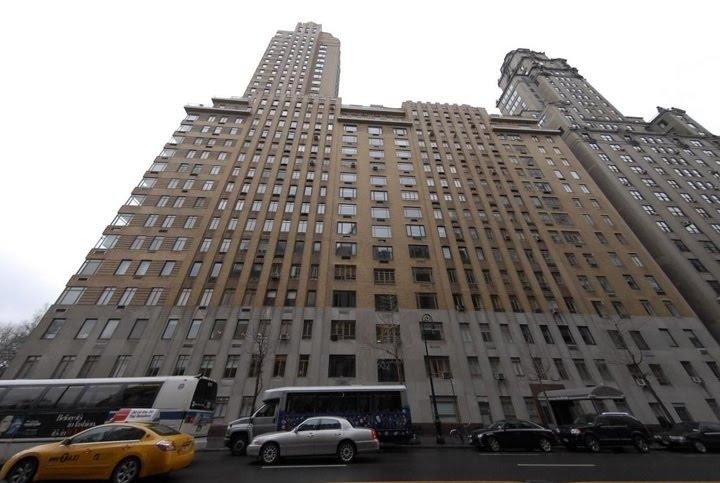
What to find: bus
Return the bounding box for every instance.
[0,374,219,467]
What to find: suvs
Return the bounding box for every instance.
[556,411,652,453]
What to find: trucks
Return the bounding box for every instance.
[222,383,417,450]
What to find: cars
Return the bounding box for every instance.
[245,415,381,467]
[655,422,720,456]
[465,417,559,451]
[0,407,195,481]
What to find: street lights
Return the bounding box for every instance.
[420,310,448,444]
